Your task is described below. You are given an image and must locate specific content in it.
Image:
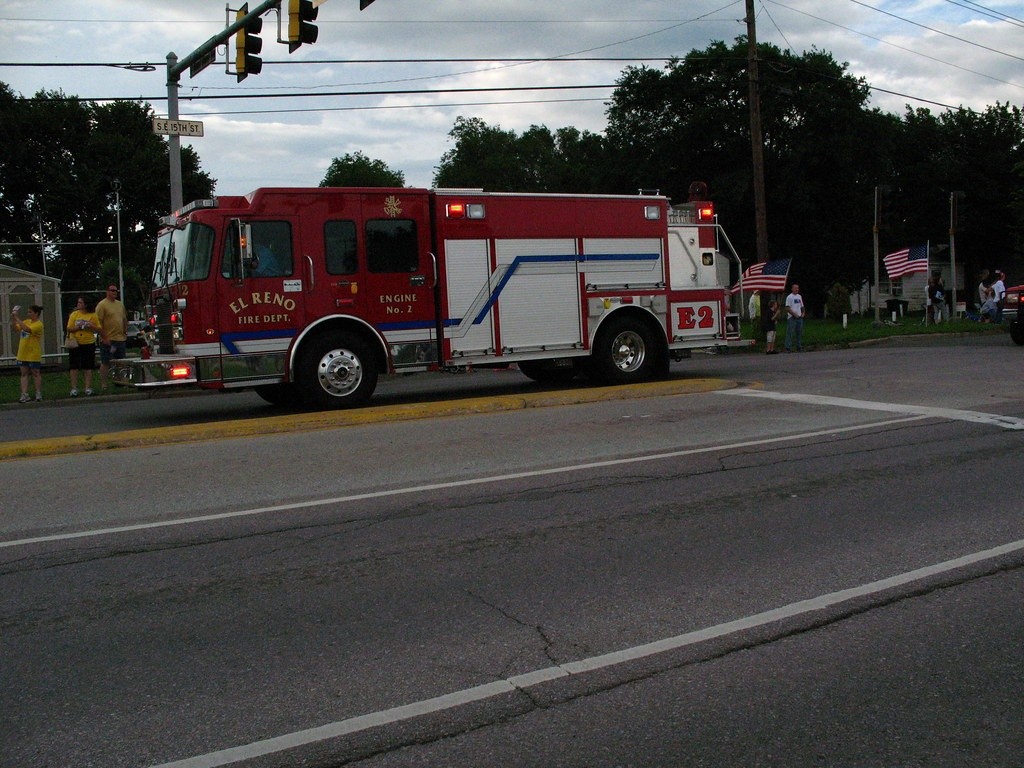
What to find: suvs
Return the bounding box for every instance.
[1000,283,1024,347]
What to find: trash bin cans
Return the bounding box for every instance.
[886,299,909,317]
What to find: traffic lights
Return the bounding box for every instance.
[288,0,319,53]
[236,2,263,76]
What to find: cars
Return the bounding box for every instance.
[125,321,150,347]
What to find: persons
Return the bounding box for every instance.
[785,284,805,352]
[96,284,129,392]
[66,296,101,398]
[749,289,761,339]
[979,269,1006,325]
[925,271,948,327]
[251,232,282,277]
[764,300,777,355]
[12,304,44,403]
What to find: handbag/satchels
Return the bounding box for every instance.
[65,336,79,349]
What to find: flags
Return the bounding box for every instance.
[730,258,791,294]
[883,242,928,279]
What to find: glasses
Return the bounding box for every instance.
[109,289,117,292]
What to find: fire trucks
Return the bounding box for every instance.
[107,185,742,413]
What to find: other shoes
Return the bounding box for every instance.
[766,350,779,354]
[70,389,78,397]
[84,388,93,395]
[19,393,31,403]
[35,392,42,401]
